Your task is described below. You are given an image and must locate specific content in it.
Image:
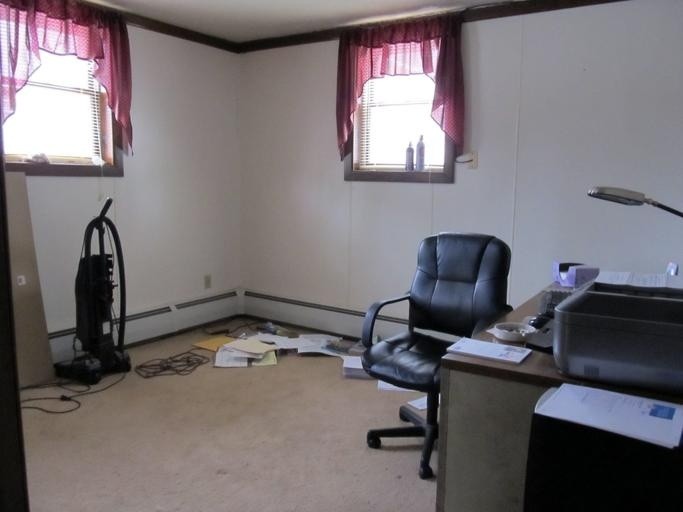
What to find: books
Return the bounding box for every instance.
[446,337,533,364]
[342,356,376,379]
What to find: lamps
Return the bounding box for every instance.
[588,186,683,218]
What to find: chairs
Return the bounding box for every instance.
[362,235,513,478]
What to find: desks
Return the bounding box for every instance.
[437,280,683,512]
